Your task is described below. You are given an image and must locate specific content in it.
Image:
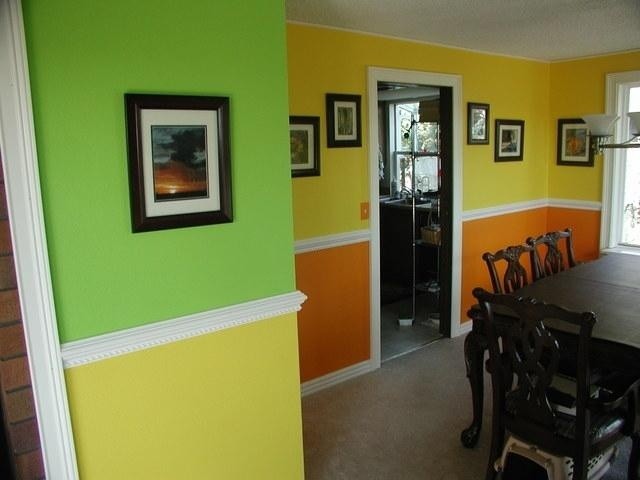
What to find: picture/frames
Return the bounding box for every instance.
[494,119,525,162]
[289,115,321,178]
[325,92,362,148]
[556,118,594,167]
[123,92,232,233]
[467,102,490,145]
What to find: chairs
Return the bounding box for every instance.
[472,287,640,480]
[481,228,578,294]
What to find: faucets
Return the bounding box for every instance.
[401,187,411,195]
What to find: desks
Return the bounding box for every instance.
[460,253,640,449]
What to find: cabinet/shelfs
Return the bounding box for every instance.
[411,114,441,323]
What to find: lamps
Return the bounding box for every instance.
[580,111,640,156]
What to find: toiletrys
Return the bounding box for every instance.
[391,176,397,195]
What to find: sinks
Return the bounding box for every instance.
[396,199,429,205]
